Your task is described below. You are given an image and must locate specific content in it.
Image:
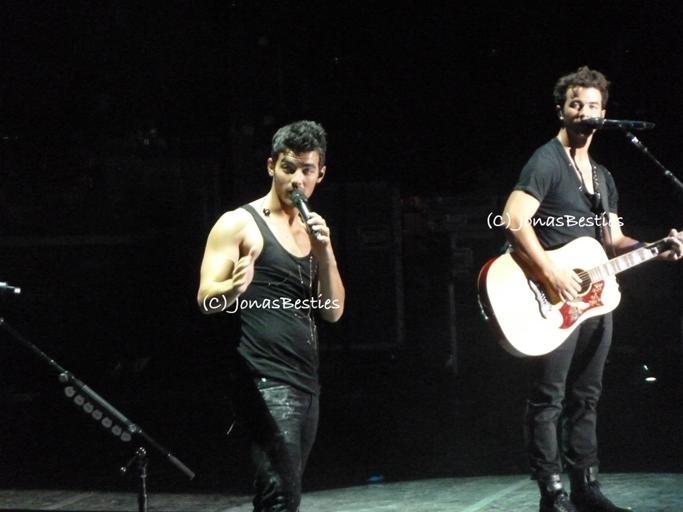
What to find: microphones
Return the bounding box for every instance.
[290,189,320,237]
[0,282,22,297]
[589,117,655,132]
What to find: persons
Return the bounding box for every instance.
[500,64,683,512]
[197,119,346,511]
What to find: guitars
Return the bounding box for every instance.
[476,231,683,357]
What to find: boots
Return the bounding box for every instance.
[568,465,633,512]
[532,471,578,512]
